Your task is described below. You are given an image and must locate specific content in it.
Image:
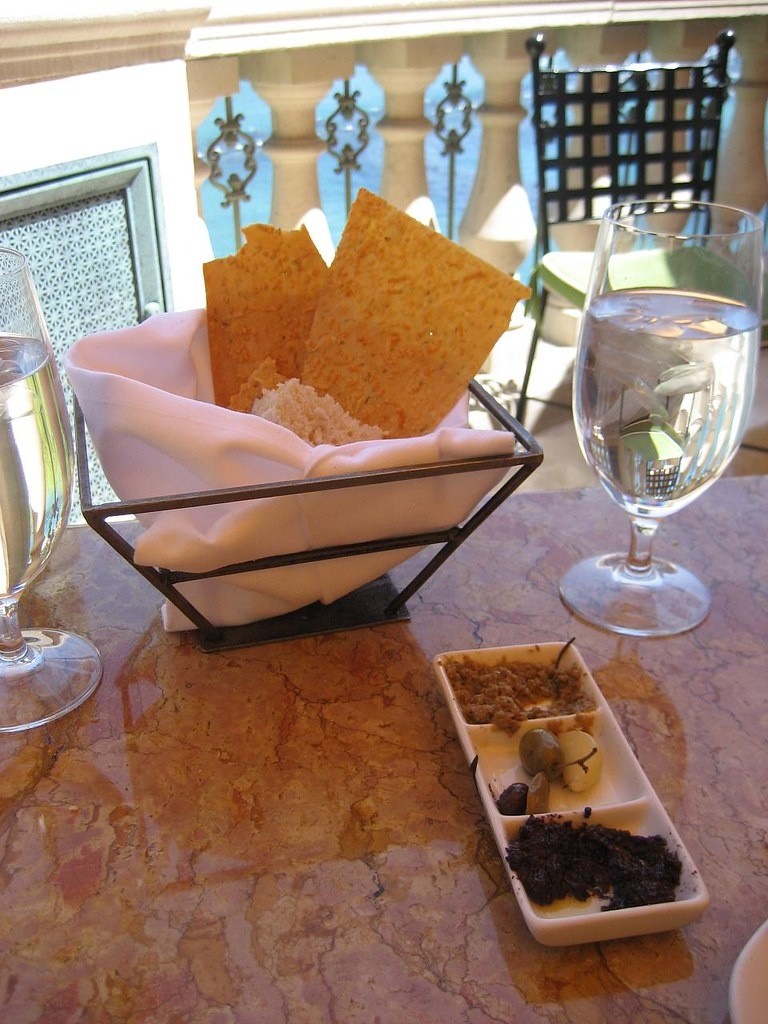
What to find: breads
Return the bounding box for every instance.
[228,362,386,450]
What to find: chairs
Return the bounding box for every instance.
[509,29,768,424]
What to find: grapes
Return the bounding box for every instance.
[518,728,565,782]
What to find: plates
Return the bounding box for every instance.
[727,920,768,1022]
[434,642,711,945]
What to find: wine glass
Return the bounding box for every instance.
[3,248,103,734]
[560,199,766,638]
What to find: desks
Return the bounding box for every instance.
[1,476,768,1024]
